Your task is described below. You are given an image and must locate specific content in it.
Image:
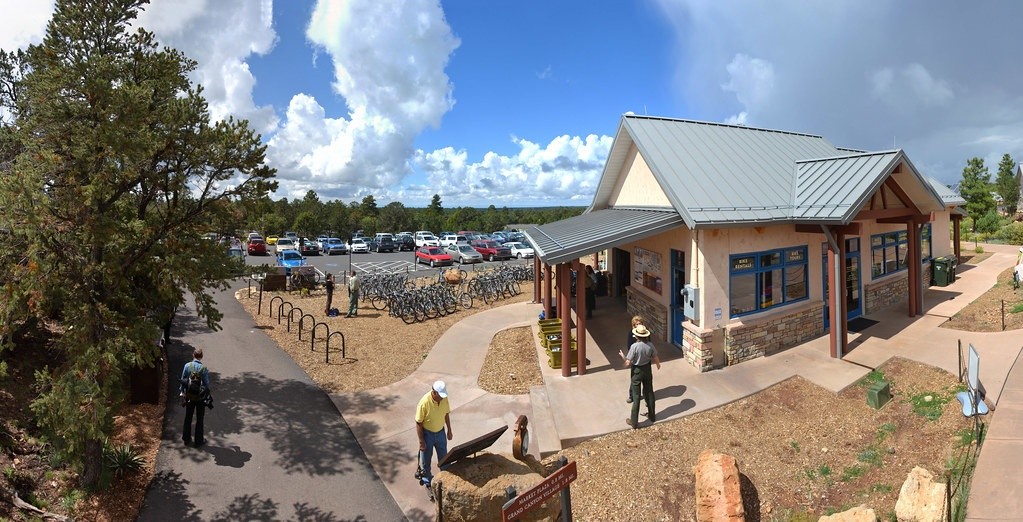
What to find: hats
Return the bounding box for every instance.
[631,325,650,337]
[432,381,449,399]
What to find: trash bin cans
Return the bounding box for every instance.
[944,256,958,283]
[929,257,951,287]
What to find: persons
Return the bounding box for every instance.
[619,325,660,429]
[626,315,650,406]
[344,271,359,318]
[585,265,598,320]
[299,236,304,254]
[325,273,335,317]
[414,381,453,500]
[178,349,208,448]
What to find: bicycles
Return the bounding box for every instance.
[352,265,557,324]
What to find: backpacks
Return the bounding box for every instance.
[187,361,207,398]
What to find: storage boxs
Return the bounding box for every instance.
[537,318,578,370]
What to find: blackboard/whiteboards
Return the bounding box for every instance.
[967,344,980,390]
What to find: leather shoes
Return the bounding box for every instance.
[626,418,637,429]
[645,414,650,419]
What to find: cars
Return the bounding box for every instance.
[274,238,296,257]
[246,232,267,256]
[274,229,536,268]
[225,237,244,256]
[275,249,308,276]
[265,233,279,245]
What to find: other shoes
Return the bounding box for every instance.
[627,398,633,403]
[184,437,192,446]
[422,473,434,482]
[195,438,207,448]
